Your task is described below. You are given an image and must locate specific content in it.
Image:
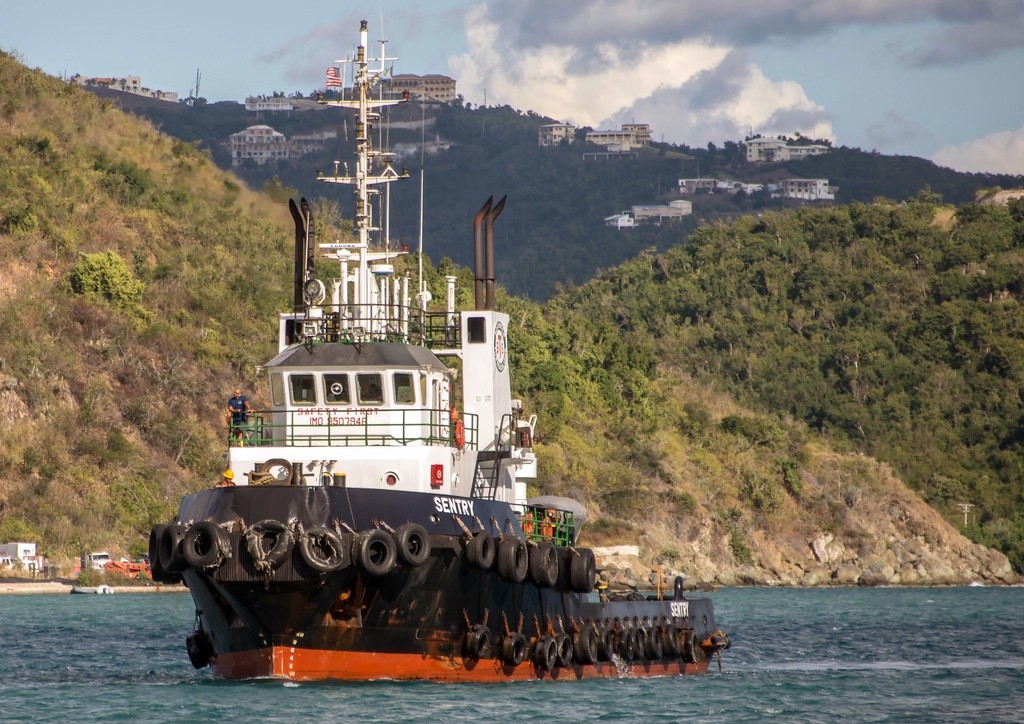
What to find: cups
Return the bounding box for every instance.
[324,474,330,486]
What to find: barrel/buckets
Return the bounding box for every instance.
[334,473,346,487]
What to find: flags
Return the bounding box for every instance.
[326,67,342,88]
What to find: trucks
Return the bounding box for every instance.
[80,551,112,576]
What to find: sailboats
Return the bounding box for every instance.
[146,15,733,686]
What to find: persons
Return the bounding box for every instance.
[541,510,556,543]
[214,470,237,487]
[522,514,535,540]
[227,389,255,448]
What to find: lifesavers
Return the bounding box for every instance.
[521,513,535,539]
[243,519,432,577]
[185,628,212,670]
[466,530,597,595]
[464,622,706,673]
[147,522,231,587]
[542,517,552,542]
[455,419,466,448]
